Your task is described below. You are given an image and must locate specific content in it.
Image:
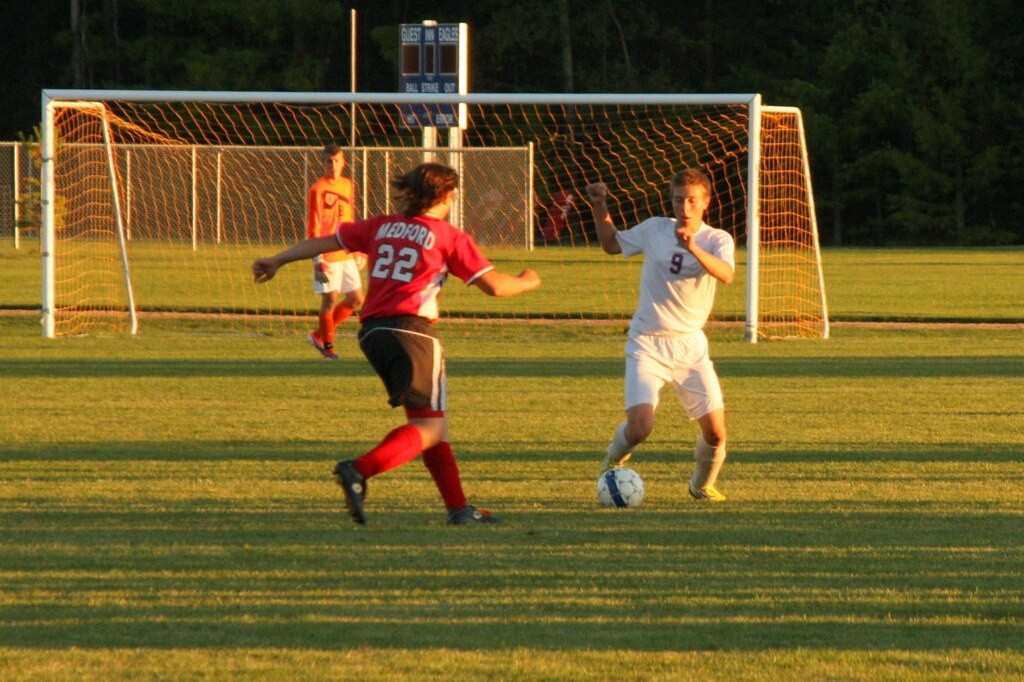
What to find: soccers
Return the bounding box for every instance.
[599,467,644,508]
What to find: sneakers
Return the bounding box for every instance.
[307,332,339,359]
[446,505,503,524]
[686,483,726,503]
[601,455,625,474]
[333,460,369,525]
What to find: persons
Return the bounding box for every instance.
[542,185,578,243]
[584,168,737,502]
[300,142,363,361]
[466,179,536,245]
[251,162,540,525]
